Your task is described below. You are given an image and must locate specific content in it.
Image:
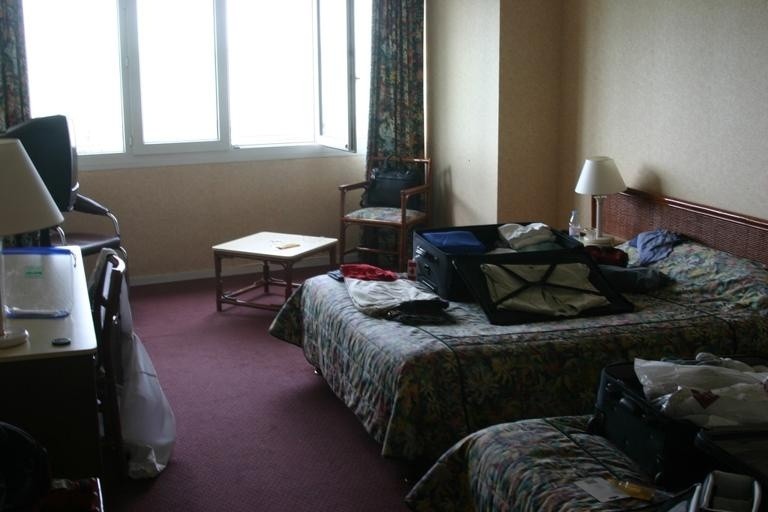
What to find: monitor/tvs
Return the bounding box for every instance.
[0,114,80,212]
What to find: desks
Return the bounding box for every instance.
[0,242,97,492]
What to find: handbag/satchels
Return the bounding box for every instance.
[360,153,420,208]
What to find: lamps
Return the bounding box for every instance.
[573,155,630,246]
[0,140,66,349]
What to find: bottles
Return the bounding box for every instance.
[567,208,581,242]
[606,476,656,502]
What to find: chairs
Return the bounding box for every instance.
[28,189,128,291]
[88,250,131,467]
[337,155,436,276]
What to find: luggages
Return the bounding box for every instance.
[584,352,767,501]
[412,221,637,325]
